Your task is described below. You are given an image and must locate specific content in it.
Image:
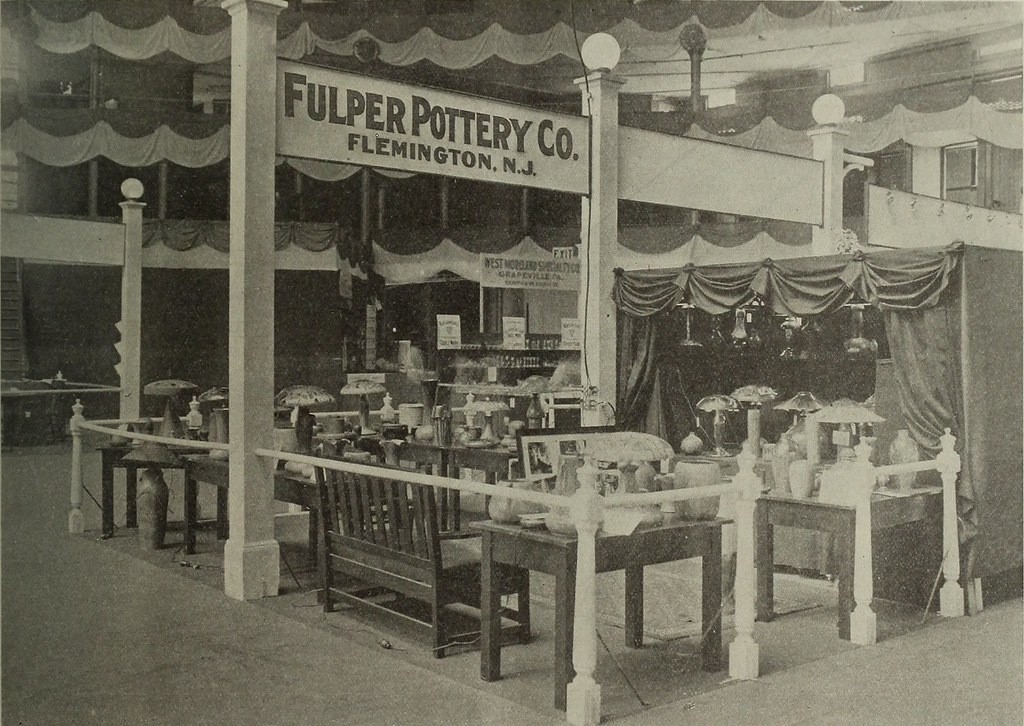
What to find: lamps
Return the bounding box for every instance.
[120,177,145,200]
[811,93,846,126]
[581,32,622,73]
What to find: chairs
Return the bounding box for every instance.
[93,377,967,684]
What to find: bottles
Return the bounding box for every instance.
[118,383,920,540]
